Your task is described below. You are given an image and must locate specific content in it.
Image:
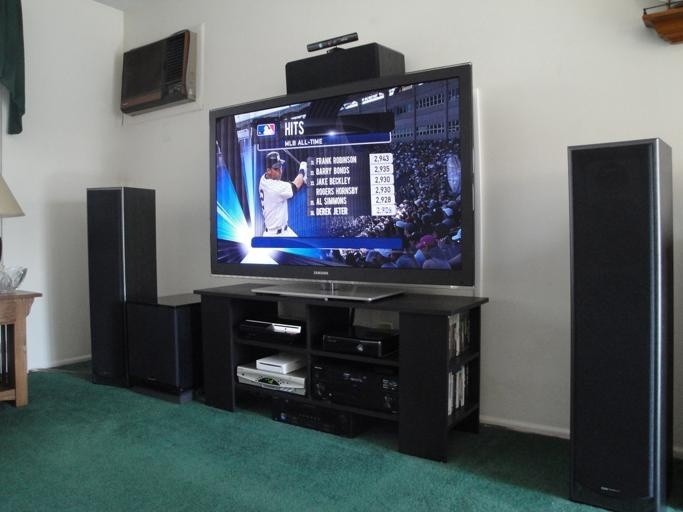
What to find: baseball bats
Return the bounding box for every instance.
[283,149,301,165]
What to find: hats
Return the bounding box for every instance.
[395,199,462,248]
[266,152,287,168]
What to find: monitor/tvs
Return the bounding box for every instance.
[209,61,484,301]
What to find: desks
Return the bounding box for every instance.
[0,290,42,407]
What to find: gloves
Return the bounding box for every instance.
[298,161,307,184]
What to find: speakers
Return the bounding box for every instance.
[286,42,405,95]
[86,186,157,388]
[567,137,673,512]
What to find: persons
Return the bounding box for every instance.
[320,139,462,271]
[258,152,307,237]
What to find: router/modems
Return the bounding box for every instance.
[256,353,305,374]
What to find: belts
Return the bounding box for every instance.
[265,226,287,235]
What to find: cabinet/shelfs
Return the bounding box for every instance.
[191,282,487,462]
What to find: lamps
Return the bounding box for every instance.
[0,174,25,218]
